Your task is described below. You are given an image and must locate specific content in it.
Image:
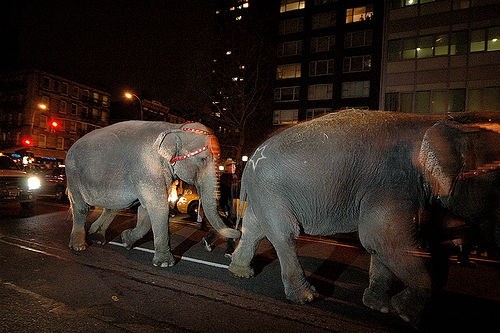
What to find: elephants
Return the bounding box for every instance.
[228,108,500,321]
[65,119,241,268]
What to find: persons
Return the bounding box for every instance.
[201,158,237,259]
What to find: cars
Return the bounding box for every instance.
[36,168,67,202]
[176,189,248,219]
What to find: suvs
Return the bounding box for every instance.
[0,155,36,208]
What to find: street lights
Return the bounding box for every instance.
[124,91,143,119]
